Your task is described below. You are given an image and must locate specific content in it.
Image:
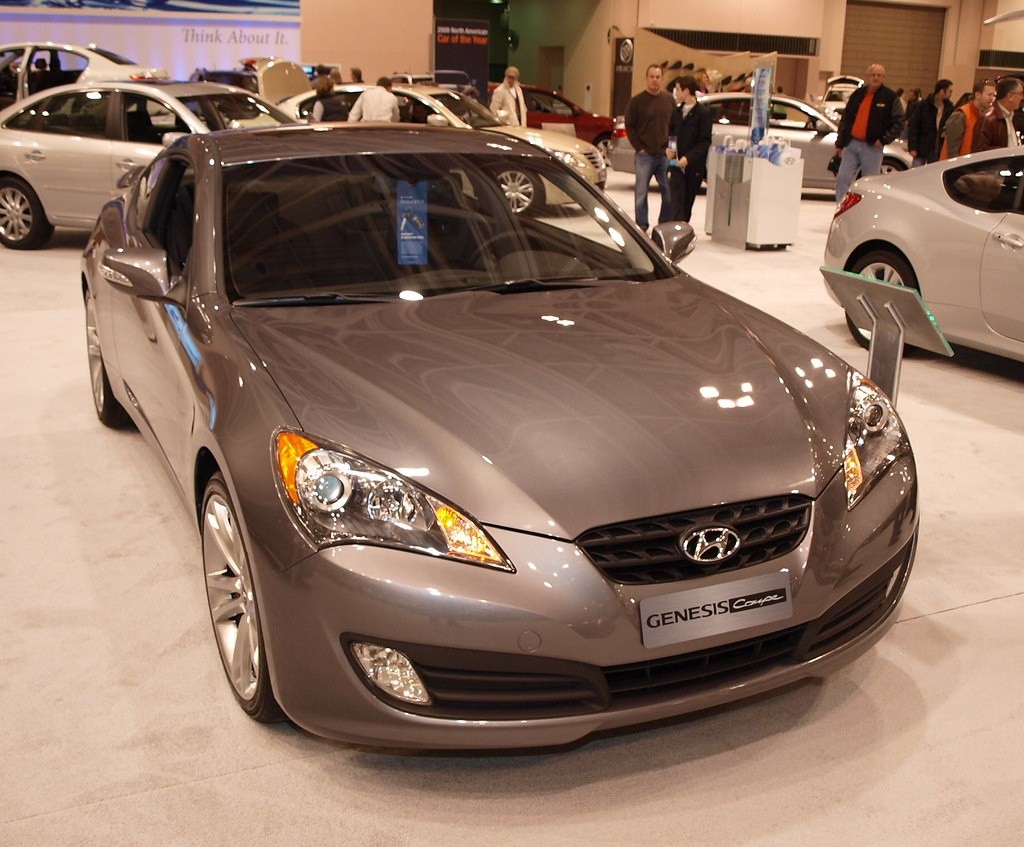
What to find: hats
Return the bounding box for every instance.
[506,67,520,79]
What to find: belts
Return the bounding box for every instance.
[852,137,866,142]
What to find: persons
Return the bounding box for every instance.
[489,66,527,127]
[624,64,677,232]
[310,64,364,88]
[771,85,787,119]
[347,77,401,123]
[715,77,752,125]
[835,63,905,205]
[695,69,711,96]
[658,75,713,221]
[897,74,1023,175]
[312,75,347,122]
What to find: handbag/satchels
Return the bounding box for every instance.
[827,154,842,177]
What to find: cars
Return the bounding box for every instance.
[225,56,608,219]
[434,69,480,122]
[486,82,615,168]
[0,78,313,250]
[0,42,174,119]
[817,76,865,127]
[608,92,914,191]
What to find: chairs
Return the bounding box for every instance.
[377,175,492,281]
[126,110,163,145]
[228,188,314,299]
[30,59,51,94]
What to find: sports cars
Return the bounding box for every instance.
[78,124,921,755]
[824,144,1024,366]
